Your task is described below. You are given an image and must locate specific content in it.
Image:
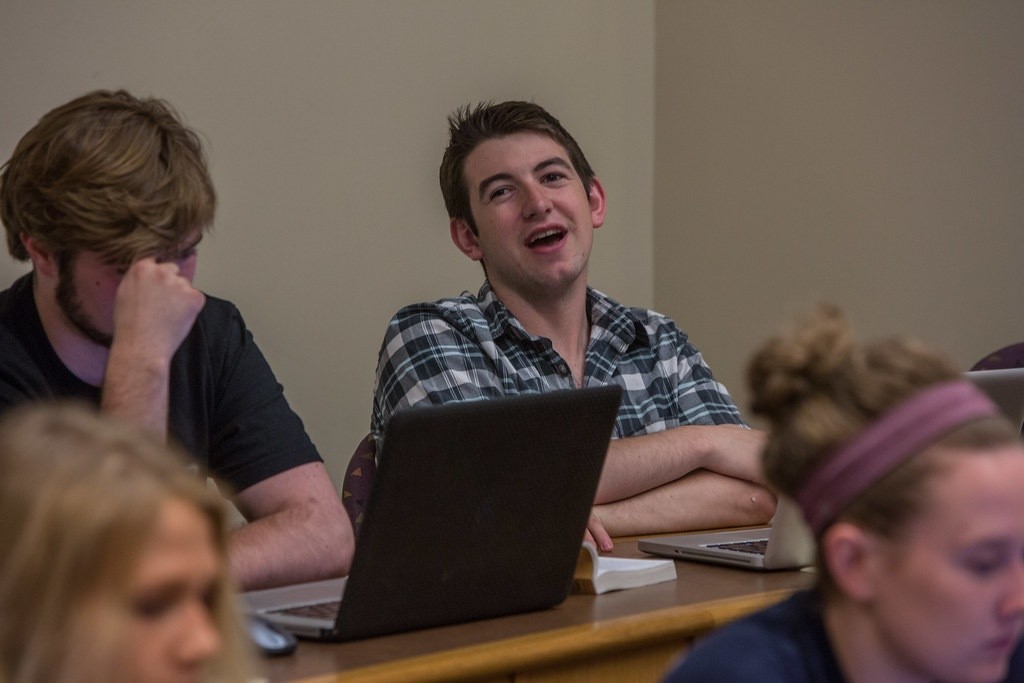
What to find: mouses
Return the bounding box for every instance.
[229,611,298,656]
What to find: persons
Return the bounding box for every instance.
[0,90,357,589]
[370,101,781,551]
[0,409,256,683]
[661,306,1024,683]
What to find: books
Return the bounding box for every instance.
[571,543,678,594]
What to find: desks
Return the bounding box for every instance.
[252,524,818,683]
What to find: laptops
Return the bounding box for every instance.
[229,385,624,644]
[637,368,1024,571]
